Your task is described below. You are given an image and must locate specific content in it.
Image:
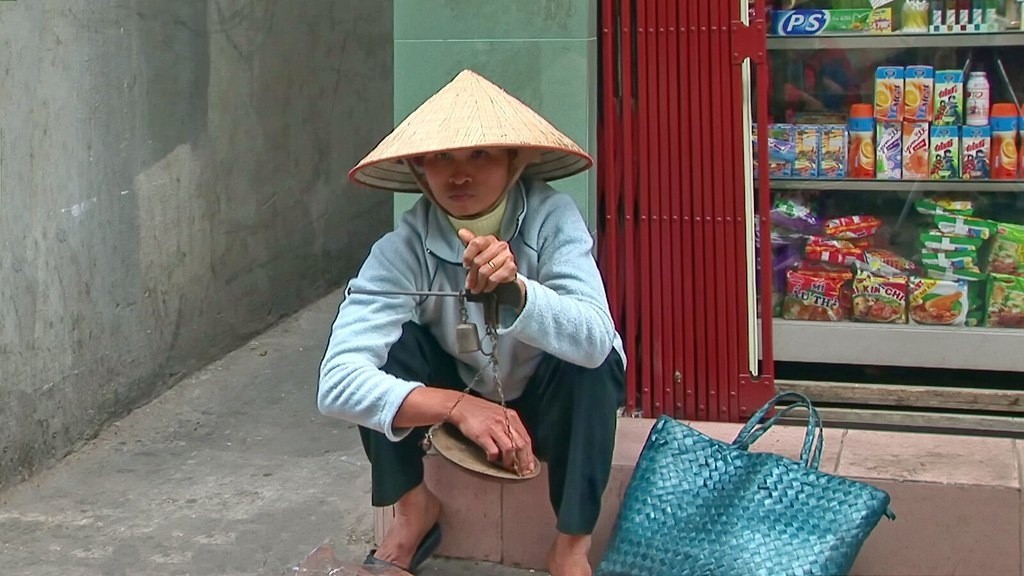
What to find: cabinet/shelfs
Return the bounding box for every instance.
[765,31,1024,375]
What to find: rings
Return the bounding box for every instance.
[488,261,495,272]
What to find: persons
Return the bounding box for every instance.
[317,71,628,576]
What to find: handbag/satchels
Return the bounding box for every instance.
[594,390,895,576]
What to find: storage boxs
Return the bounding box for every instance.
[752,119,849,181]
[770,8,892,36]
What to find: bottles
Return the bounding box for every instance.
[990,103,1018,178]
[1018,104,1024,178]
[966,71,989,126]
[847,103,876,179]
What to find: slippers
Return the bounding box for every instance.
[354,525,442,576]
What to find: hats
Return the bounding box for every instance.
[348,69,593,193]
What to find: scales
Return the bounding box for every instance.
[345,285,543,483]
